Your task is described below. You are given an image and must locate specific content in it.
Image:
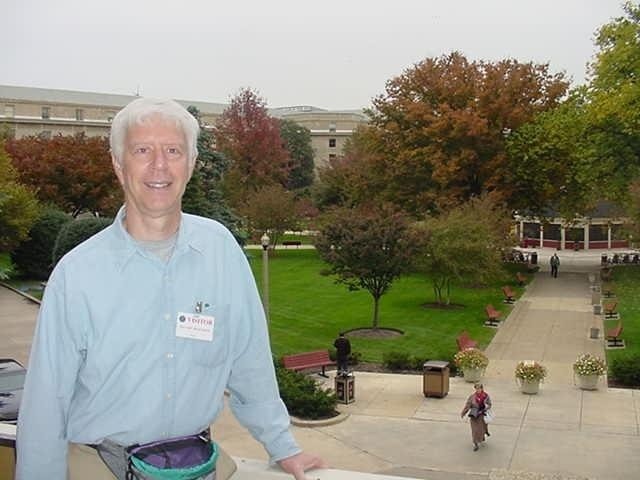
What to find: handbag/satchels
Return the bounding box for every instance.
[97,428,218,479]
[483,409,495,424]
[469,409,478,418]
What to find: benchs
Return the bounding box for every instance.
[283,350,336,378]
[516,272,527,288]
[503,285,516,304]
[603,280,617,299]
[485,304,502,326]
[283,241,301,248]
[603,300,618,319]
[608,322,624,347]
[456,330,479,349]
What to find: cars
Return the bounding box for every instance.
[0,354,30,429]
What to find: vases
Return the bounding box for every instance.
[521,382,539,395]
[579,376,600,391]
[464,369,480,382]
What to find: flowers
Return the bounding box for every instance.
[573,352,608,376]
[453,348,489,376]
[514,360,548,385]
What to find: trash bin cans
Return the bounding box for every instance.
[424,360,450,397]
[335,371,355,403]
[531,252,537,264]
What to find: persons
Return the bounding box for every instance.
[460,383,495,452]
[334,331,352,375]
[9,90,331,479]
[550,253,560,278]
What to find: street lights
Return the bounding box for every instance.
[259,231,270,324]
